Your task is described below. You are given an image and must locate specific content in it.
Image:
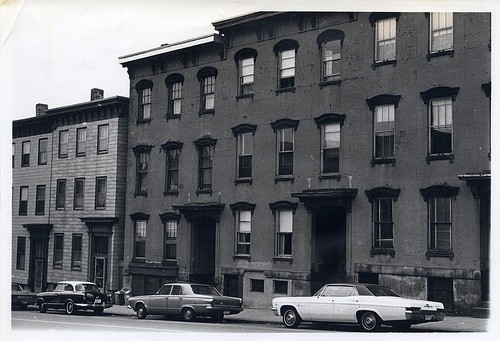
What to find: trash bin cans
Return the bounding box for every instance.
[124,291,132,306]
[115,291,124,305]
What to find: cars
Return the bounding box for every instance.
[129,283,244,322]
[272,282,446,331]
[36,280,113,314]
[12,283,36,310]
